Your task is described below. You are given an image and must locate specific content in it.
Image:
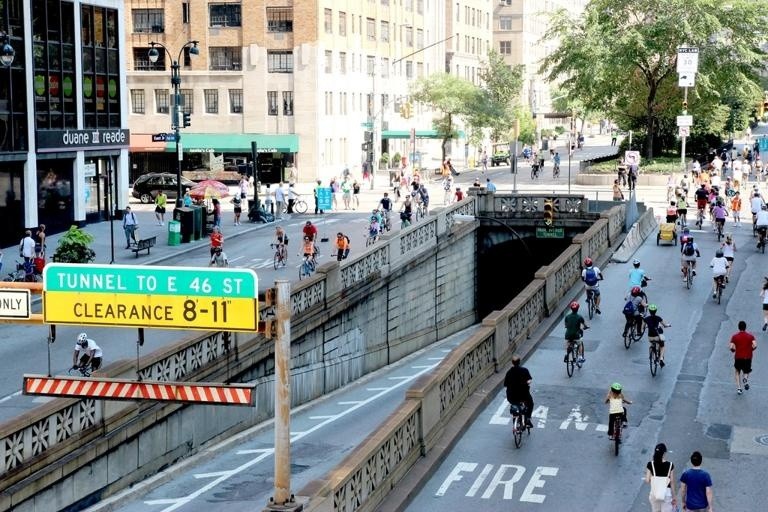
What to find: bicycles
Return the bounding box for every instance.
[312,244,320,254]
[622,278,671,378]
[364,182,453,248]
[511,379,536,449]
[564,325,592,378]
[68,364,93,377]
[331,254,345,261]
[668,150,763,203]
[530,164,539,180]
[270,244,288,270]
[297,254,320,280]
[607,399,630,455]
[752,212,768,255]
[282,197,308,214]
[552,161,560,179]
[585,288,597,318]
[679,198,725,305]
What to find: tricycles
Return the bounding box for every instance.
[656,221,678,246]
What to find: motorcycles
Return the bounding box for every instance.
[2,256,39,282]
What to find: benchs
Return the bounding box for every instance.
[131,236,159,259]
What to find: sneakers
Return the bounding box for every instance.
[659,357,665,367]
[737,388,744,394]
[577,358,586,363]
[595,306,601,313]
[524,419,533,427]
[762,323,768,331]
[692,270,696,276]
[743,378,749,390]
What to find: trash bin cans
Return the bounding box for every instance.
[248,199,261,221]
[168,205,208,246]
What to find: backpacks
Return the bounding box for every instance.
[623,298,636,317]
[584,267,597,286]
[650,461,671,501]
[684,243,694,256]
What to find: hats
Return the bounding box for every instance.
[648,304,657,311]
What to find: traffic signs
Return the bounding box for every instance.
[0,289,30,318]
[42,262,260,333]
[536,228,565,239]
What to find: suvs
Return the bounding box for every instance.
[491,150,511,166]
[132,172,199,204]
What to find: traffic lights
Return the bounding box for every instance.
[184,113,191,127]
[408,104,414,118]
[682,102,687,111]
[400,103,408,119]
[543,200,553,225]
[760,103,768,116]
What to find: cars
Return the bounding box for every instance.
[522,148,536,159]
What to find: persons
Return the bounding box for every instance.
[271,221,350,276]
[728,320,757,394]
[760,282,768,331]
[521,132,638,201]
[230,167,360,227]
[564,302,588,362]
[665,125,768,299]
[73,333,103,372]
[639,305,671,367]
[580,258,604,314]
[441,150,495,205]
[644,443,677,512]
[622,287,648,336]
[504,355,534,427]
[210,225,228,268]
[362,158,429,244]
[35,224,46,259]
[155,190,167,226]
[122,206,138,249]
[605,382,632,440]
[625,258,651,299]
[19,231,36,263]
[681,450,712,512]
[184,188,220,227]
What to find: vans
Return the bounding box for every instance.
[225,156,248,167]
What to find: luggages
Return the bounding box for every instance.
[34,251,45,274]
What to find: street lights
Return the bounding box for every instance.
[453,213,538,271]
[148,40,199,218]
[0,29,15,69]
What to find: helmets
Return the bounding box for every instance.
[687,237,693,243]
[584,258,592,266]
[570,302,580,311]
[77,332,87,344]
[716,250,723,257]
[631,286,640,296]
[215,246,222,252]
[611,382,623,391]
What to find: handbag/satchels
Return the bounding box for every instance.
[658,333,667,341]
[510,404,525,415]
[20,245,24,257]
